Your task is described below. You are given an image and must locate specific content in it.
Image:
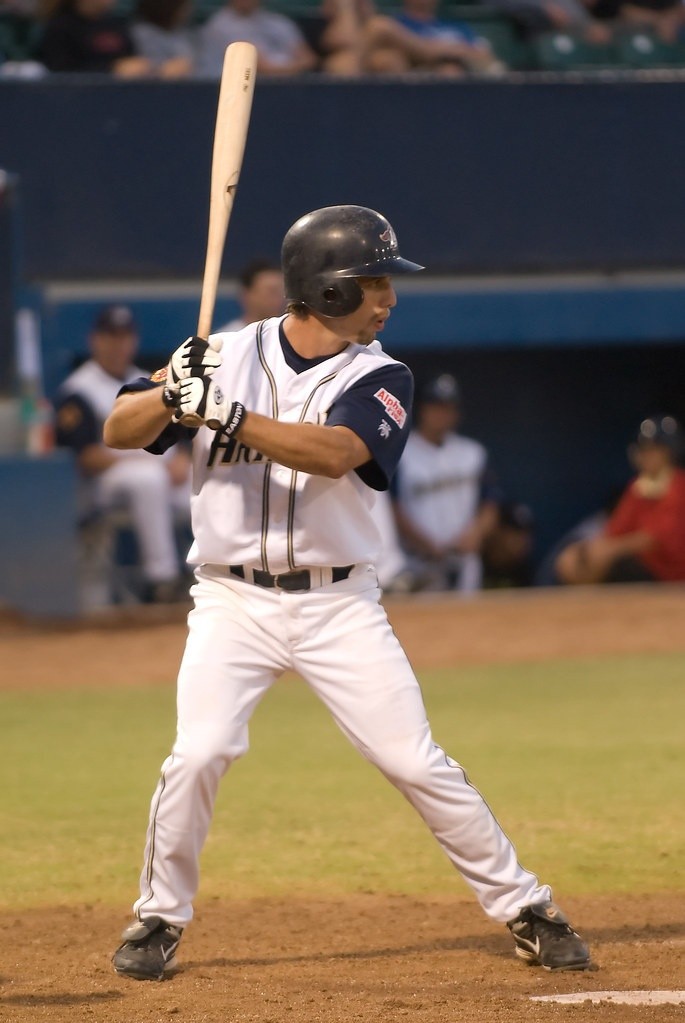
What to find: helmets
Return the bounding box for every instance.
[280,205,425,318]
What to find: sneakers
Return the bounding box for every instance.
[110,917,184,981]
[507,900,591,971]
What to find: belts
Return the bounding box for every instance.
[230,564,354,590]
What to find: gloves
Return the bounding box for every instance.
[162,336,223,405]
[171,375,232,428]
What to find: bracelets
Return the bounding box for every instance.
[222,402,245,438]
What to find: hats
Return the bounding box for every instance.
[98,305,135,335]
[420,378,458,403]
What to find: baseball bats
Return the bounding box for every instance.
[178,40,259,430]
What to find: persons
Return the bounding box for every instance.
[102,205,597,979]
[21,0,685,76]
[391,371,506,594]
[57,305,193,604]
[214,262,285,331]
[557,428,685,581]
